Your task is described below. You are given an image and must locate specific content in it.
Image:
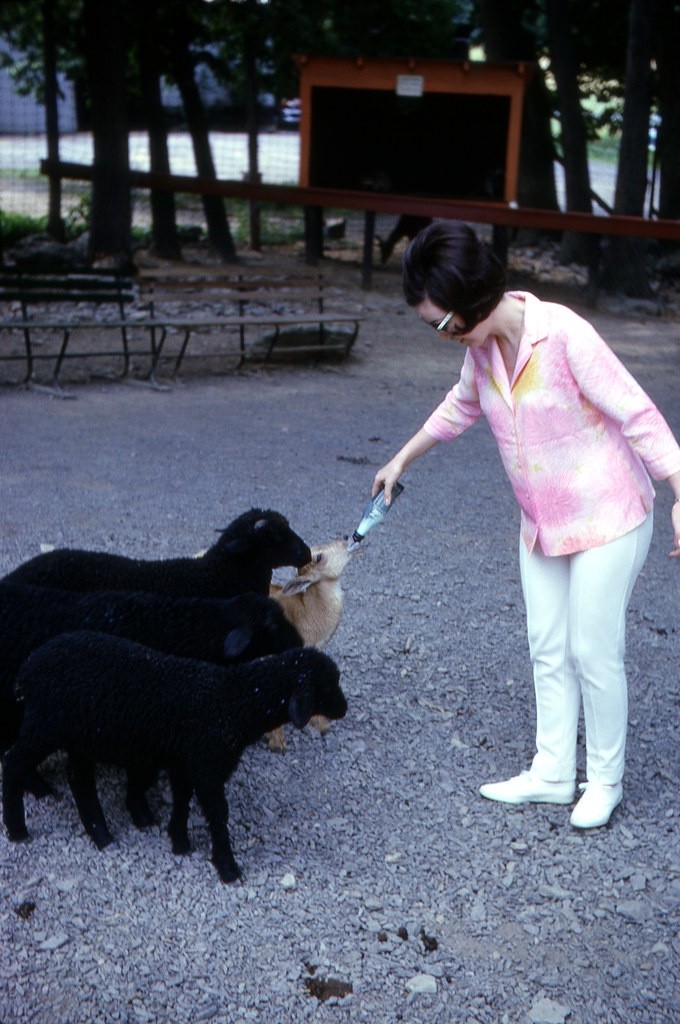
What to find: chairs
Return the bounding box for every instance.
[0,265,365,388]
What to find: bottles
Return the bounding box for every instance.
[352,481,405,543]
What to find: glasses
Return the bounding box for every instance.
[420,309,454,336]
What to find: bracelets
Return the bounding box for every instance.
[674,498,679,503]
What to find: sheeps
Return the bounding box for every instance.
[0,506,360,883]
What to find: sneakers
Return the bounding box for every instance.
[479,770,576,804]
[571,781,622,827]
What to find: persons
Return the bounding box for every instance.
[371,220,680,828]
